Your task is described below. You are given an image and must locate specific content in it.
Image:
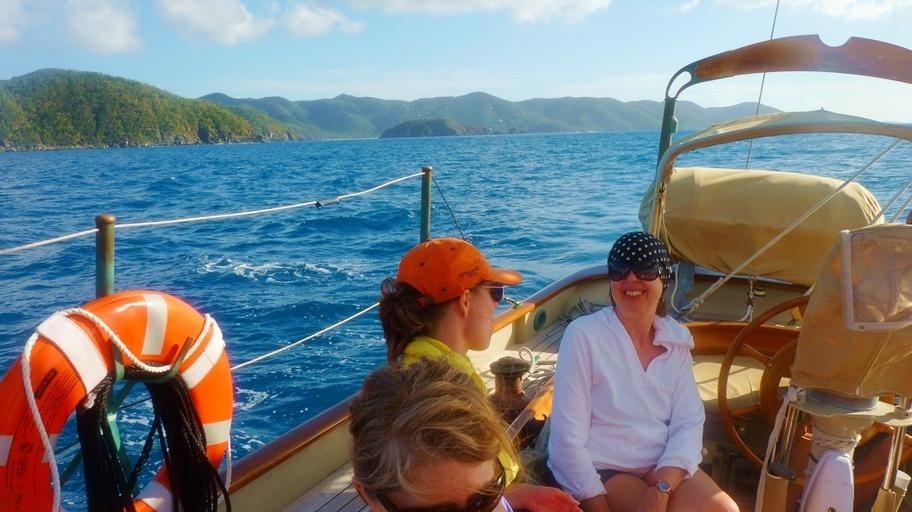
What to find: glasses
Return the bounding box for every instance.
[376,457,506,512]
[480,284,503,301]
[608,260,663,280]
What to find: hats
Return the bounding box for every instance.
[608,232,671,287]
[397,239,521,304]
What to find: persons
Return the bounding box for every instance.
[347,357,523,511]
[378,238,583,512]
[546,232,739,511]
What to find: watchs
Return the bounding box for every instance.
[648,480,677,497]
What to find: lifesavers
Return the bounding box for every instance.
[1,292,232,510]
